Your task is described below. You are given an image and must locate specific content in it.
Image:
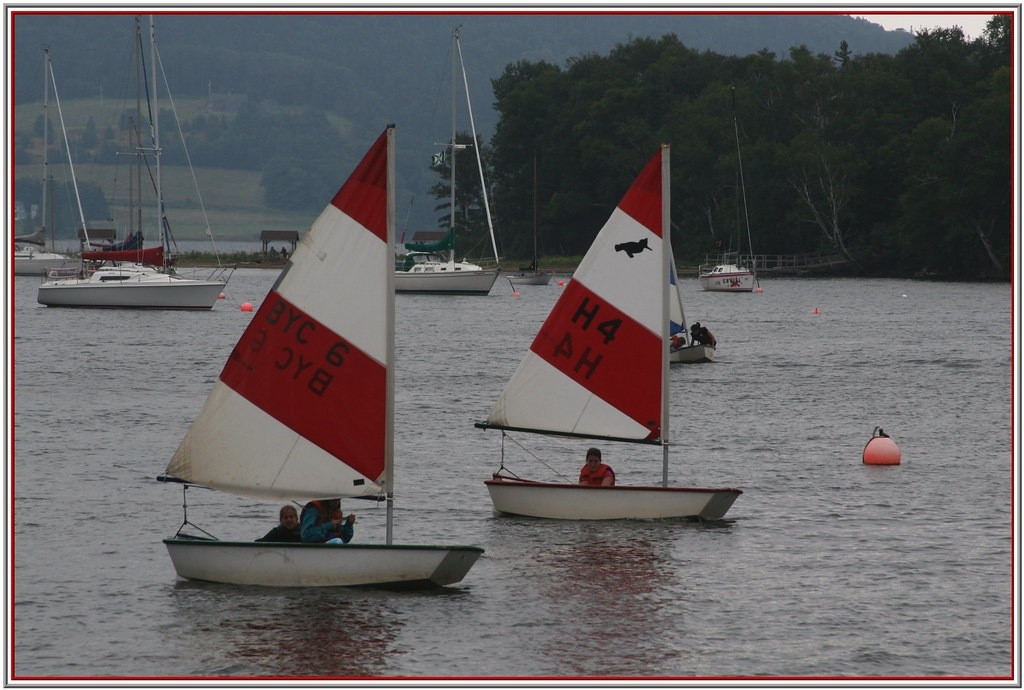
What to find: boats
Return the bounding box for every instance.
[698,253,757,293]
[505,269,554,286]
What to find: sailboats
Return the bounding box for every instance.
[14,36,91,277]
[394,24,503,296]
[669,237,719,365]
[472,139,744,525]
[156,121,486,587]
[80,14,166,271]
[36,14,228,313]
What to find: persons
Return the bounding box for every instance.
[669,322,716,351]
[579,449,615,486]
[255,497,357,544]
